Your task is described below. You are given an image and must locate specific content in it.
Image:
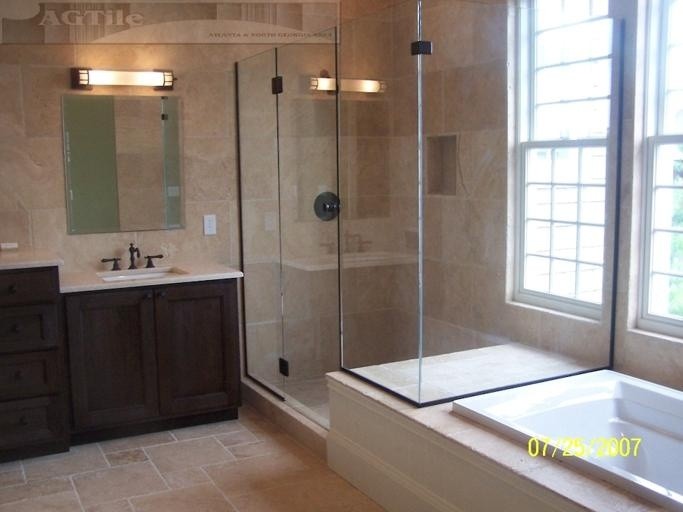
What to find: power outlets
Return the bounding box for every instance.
[204,215,217,235]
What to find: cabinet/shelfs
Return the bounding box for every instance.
[67,282,238,428]
[0,270,69,463]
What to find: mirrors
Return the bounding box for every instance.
[60,95,186,235]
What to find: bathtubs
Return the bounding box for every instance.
[454,367,682,510]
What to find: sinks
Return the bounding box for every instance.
[96,266,188,282]
[278,249,427,288]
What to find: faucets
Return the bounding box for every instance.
[132,247,141,258]
[344,230,376,254]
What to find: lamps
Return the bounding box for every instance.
[308,77,389,94]
[70,66,177,91]
